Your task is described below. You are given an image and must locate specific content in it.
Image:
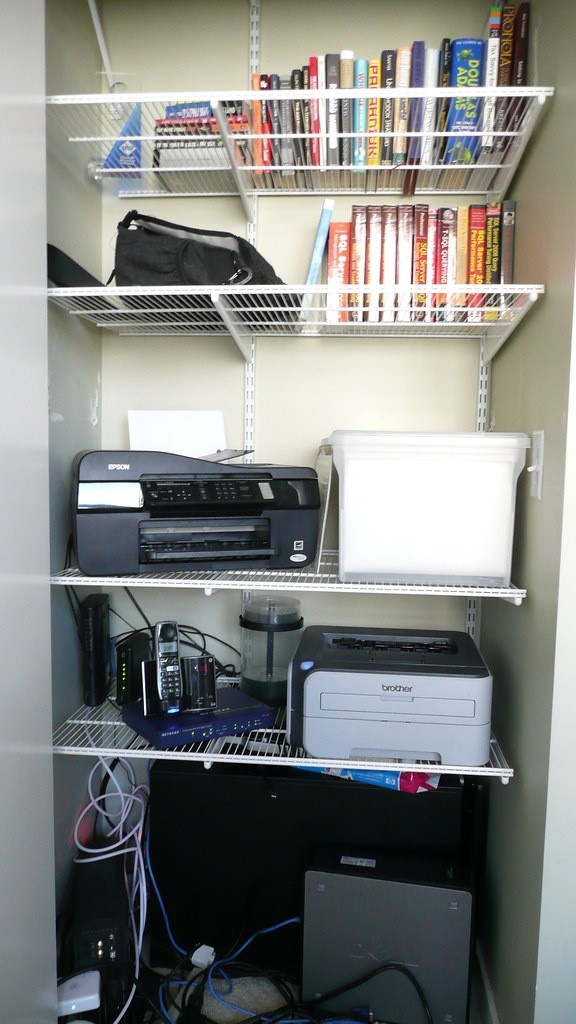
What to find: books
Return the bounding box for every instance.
[155,0,532,322]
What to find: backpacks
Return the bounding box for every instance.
[114,205,305,331]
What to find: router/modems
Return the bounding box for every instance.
[57,970,101,1016]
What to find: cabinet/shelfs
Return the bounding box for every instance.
[0,0,576,1024]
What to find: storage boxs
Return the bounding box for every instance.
[324,426,533,593]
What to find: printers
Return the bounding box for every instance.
[72,445,321,574]
[286,624,492,766]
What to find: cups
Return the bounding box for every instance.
[239,594,303,705]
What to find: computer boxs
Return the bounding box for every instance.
[148,760,464,972]
